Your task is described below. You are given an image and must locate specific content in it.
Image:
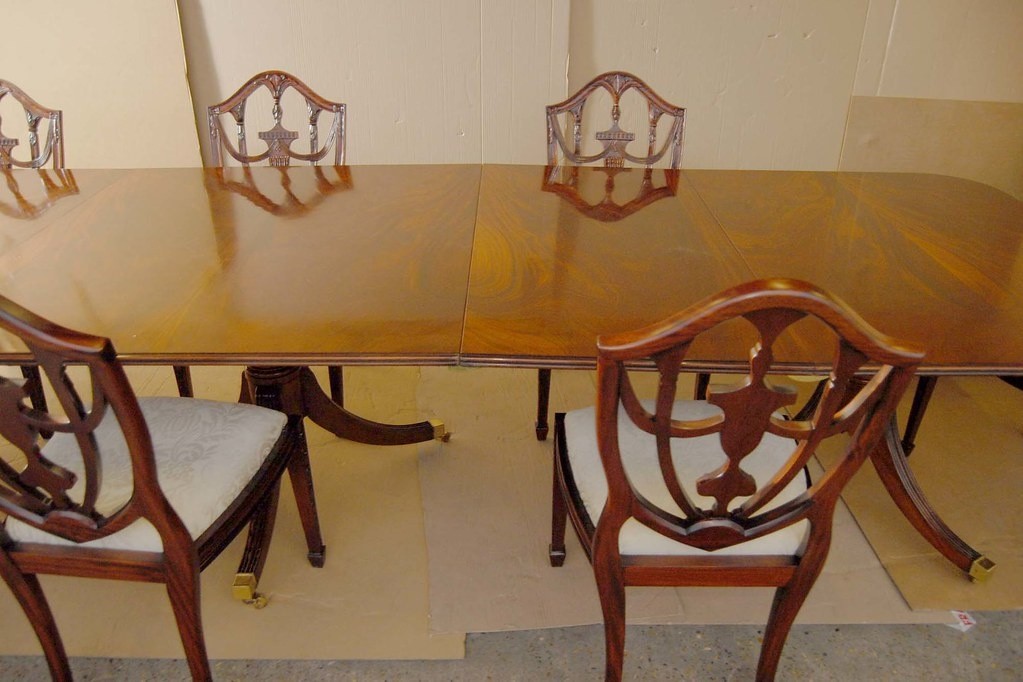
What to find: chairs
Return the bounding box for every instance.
[533,71,688,442]
[173,70,347,406]
[546,275,928,682]
[0,78,65,169]
[0,294,296,682]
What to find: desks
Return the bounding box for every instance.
[0,167,1023,611]
